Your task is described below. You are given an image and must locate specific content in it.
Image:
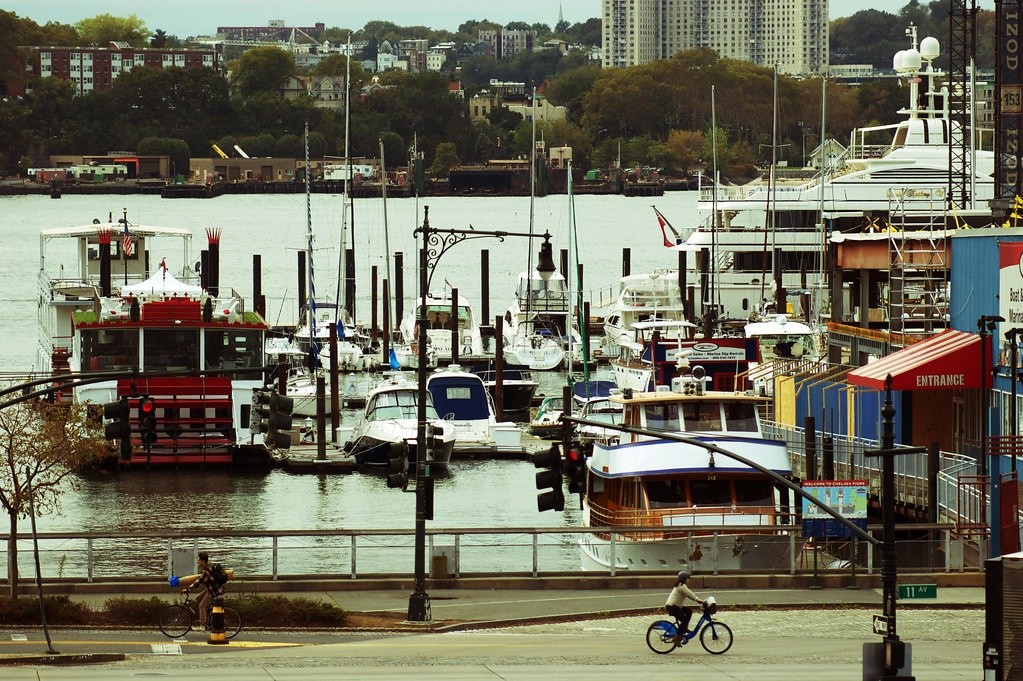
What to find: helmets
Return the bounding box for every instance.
[679,571,690,579]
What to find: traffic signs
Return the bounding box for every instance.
[872,615,888,636]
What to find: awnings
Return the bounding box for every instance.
[847,328,992,390]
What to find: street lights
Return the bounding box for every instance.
[408,206,556,620]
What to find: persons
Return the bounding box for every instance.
[180,551,224,631]
[665,571,704,647]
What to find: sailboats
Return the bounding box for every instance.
[271,29,801,466]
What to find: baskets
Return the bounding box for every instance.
[707,603,717,614]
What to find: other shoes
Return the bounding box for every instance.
[685,628,692,634]
[670,635,682,648]
[195,620,206,627]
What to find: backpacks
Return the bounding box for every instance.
[203,563,229,586]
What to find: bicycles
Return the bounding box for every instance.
[646,600,733,655]
[158,590,242,639]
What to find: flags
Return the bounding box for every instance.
[336,319,345,337]
[656,213,674,248]
[123,225,132,256]
[389,349,400,370]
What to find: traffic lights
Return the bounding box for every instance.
[534,446,565,512]
[426,425,444,463]
[138,397,157,443]
[567,442,586,493]
[386,441,409,487]
[104,399,131,460]
[266,392,294,449]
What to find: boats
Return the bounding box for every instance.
[580,298,807,573]
[24,203,279,478]
[618,21,1019,330]
